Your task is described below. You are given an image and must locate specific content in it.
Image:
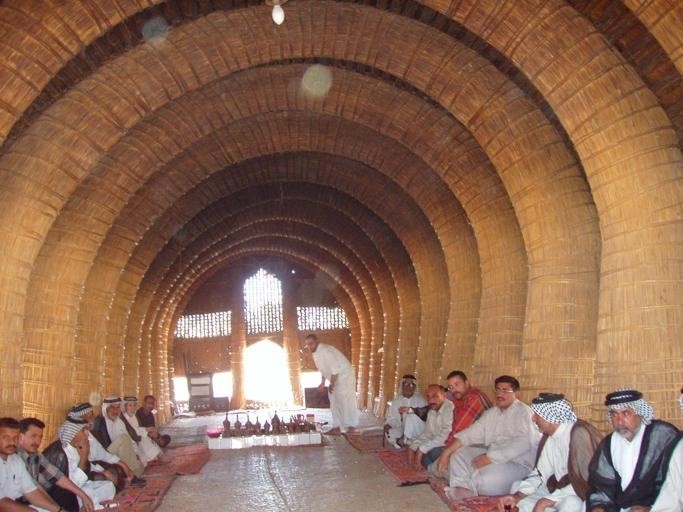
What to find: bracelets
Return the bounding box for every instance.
[328,382,333,385]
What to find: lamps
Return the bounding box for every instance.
[264,1,289,26]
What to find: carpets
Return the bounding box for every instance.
[92,474,180,512]
[342,430,520,512]
[140,440,215,476]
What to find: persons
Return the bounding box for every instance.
[305,333,358,442]
[0,395,171,512]
[384,368,683,512]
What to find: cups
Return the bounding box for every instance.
[314,422,321,432]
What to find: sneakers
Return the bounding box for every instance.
[129,476,146,488]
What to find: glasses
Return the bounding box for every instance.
[447,382,462,391]
[494,390,514,394]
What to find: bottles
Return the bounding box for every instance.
[306,414,314,424]
